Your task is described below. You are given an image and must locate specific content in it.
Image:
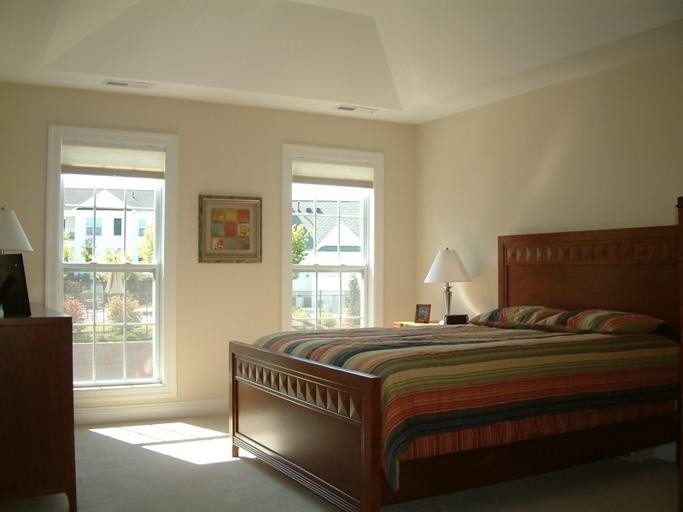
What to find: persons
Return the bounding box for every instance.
[418,308,426,320]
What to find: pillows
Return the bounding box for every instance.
[535,308,667,336]
[469,305,565,330]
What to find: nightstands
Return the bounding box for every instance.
[393,321,439,328]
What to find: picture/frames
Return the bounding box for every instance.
[415,304,431,323]
[198,195,262,263]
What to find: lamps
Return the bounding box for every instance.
[0,207,34,253]
[424,247,472,325]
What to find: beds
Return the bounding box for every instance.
[229,195,683,512]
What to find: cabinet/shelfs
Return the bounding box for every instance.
[1,300,79,511]
